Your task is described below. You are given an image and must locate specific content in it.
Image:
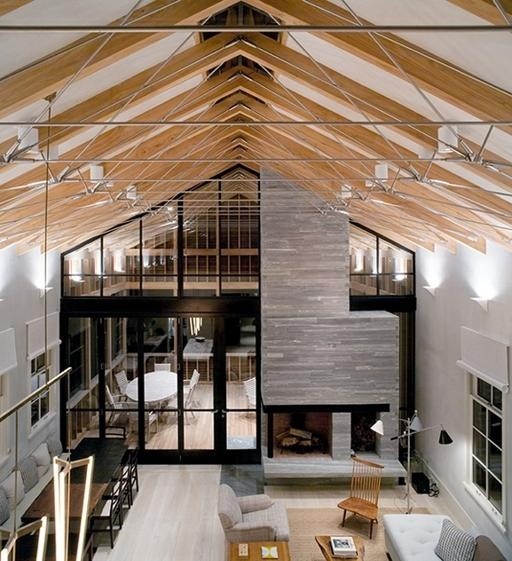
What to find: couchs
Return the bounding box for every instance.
[0,432,72,541]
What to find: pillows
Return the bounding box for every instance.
[0,432,63,526]
[434,519,477,561]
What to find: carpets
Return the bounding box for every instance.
[219,436,264,497]
[225,507,431,561]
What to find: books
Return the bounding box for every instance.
[331,537,356,556]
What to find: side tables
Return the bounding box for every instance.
[228,541,291,561]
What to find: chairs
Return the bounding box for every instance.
[105,363,200,444]
[242,377,256,418]
[383,514,508,561]
[337,457,384,539]
[217,483,290,544]
[90,426,139,548]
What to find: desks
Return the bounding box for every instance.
[21,437,135,544]
[226,345,257,384]
[183,338,213,381]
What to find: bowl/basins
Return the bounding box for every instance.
[195,337,205,342]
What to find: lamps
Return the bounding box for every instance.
[422,286,436,295]
[370,410,453,514]
[391,278,405,287]
[72,279,86,289]
[471,297,488,311]
[341,126,458,199]
[17,128,137,201]
[40,287,54,298]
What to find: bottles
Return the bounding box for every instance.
[149,327,153,336]
